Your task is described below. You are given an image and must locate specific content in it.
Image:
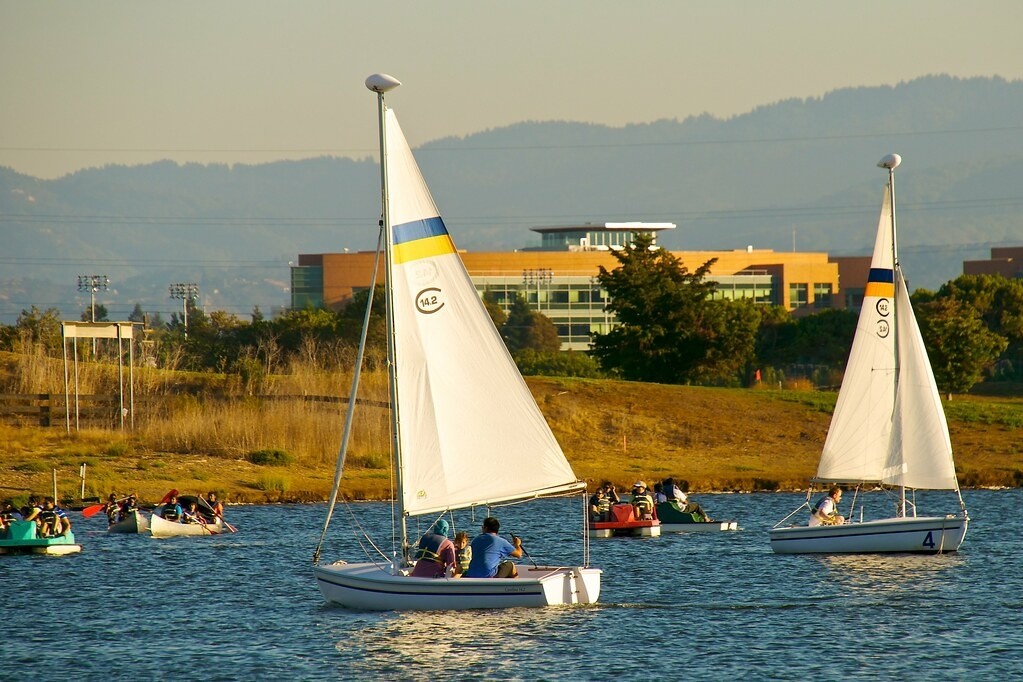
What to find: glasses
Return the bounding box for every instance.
[603,486,610,490]
[43,502,49,505]
[596,492,603,495]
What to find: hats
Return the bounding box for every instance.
[434,519,449,538]
[632,480,647,489]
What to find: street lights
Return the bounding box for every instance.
[523,269,553,313]
[168,283,198,340]
[75,274,109,363]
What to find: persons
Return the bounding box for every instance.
[467,517,522,579]
[808,487,844,527]
[104,490,223,525]
[412,519,457,579]
[453,530,473,575]
[588,477,715,523]
[0,496,71,540]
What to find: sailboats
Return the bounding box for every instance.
[310,73,605,615]
[767,154,971,556]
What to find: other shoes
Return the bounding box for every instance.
[709,518,716,522]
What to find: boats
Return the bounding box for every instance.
[107,510,149,534]
[659,522,737,531]
[584,501,661,539]
[151,513,223,537]
[0,519,82,557]
